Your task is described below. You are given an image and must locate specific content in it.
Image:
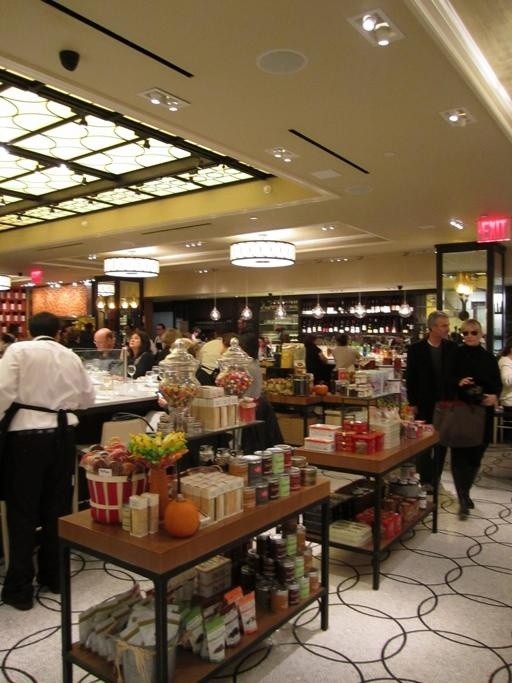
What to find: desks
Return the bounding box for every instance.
[185,420,265,451]
[58,478,333,682]
[295,425,441,590]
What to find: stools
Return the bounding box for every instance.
[492,403,510,446]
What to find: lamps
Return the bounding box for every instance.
[398,254,415,318]
[362,13,377,32]
[209,268,222,321]
[240,268,254,322]
[301,263,328,321]
[102,256,162,277]
[97,284,115,296]
[0,274,13,291]
[349,258,368,321]
[275,297,288,319]
[375,22,390,46]
[455,271,472,322]
[228,239,295,269]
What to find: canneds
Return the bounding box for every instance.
[302,465,318,486]
[288,579,300,606]
[311,569,320,594]
[300,575,309,600]
[275,443,292,467]
[279,474,291,496]
[243,486,256,510]
[239,524,315,610]
[243,454,263,479]
[290,470,302,490]
[255,450,273,476]
[228,460,248,480]
[292,455,307,468]
[252,479,270,505]
[271,585,289,615]
[270,478,280,500]
[267,447,284,474]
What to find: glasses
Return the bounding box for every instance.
[462,331,480,337]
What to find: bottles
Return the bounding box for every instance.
[302,319,414,334]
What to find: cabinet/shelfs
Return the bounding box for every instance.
[435,242,508,357]
[0,285,29,339]
[300,289,437,338]
[258,295,300,357]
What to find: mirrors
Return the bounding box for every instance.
[117,276,143,341]
[93,276,117,333]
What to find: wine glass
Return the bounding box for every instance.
[152,365,159,381]
[126,364,135,383]
[145,371,154,390]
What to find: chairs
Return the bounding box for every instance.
[72,416,148,514]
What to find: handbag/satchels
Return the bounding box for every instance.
[432,399,487,450]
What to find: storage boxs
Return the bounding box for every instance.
[276,412,320,445]
[355,369,383,398]
[380,364,396,379]
[85,470,152,526]
[384,379,403,394]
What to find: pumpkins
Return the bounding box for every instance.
[164,493,200,538]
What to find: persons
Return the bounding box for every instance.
[0,312,96,612]
[495,336,512,444]
[405,311,459,495]
[1,320,361,464]
[441,318,503,517]
